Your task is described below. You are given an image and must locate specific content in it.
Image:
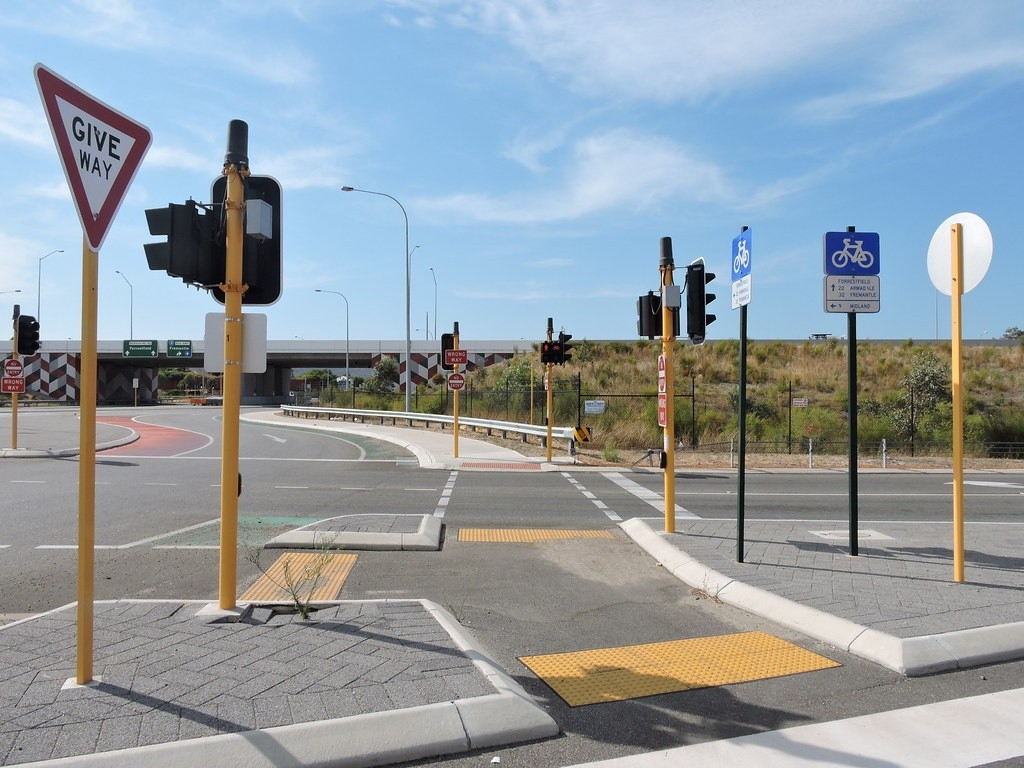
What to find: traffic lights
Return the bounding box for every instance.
[560,331,572,364]
[17,316,40,357]
[541,342,561,364]
[688,257,716,346]
[145,202,197,280]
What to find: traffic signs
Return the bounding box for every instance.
[122,340,158,359]
[167,340,193,358]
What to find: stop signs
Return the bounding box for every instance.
[447,373,466,391]
[658,355,668,392]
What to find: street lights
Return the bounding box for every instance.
[341,186,410,410]
[38,249,64,321]
[409,245,437,340]
[315,290,348,391]
[115,270,133,340]
